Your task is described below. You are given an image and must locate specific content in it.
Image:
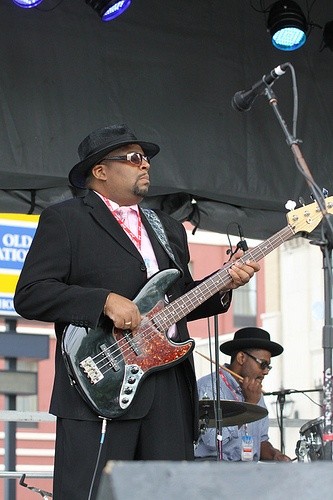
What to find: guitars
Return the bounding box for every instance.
[62,188,333,419]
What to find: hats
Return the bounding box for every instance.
[67,121,161,189]
[220,327,283,357]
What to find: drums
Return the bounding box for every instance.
[299,415,333,462]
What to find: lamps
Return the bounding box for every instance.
[11,0,131,22]
[267,0,309,51]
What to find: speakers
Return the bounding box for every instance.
[94,459,333,500]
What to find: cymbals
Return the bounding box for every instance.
[198,398,269,428]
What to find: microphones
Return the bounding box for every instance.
[231,63,288,114]
[238,224,249,255]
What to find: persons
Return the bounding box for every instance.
[13,123,261,500]
[193,327,292,463]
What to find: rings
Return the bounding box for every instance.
[124,322,132,326]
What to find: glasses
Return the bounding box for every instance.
[242,351,272,371]
[103,151,151,167]
